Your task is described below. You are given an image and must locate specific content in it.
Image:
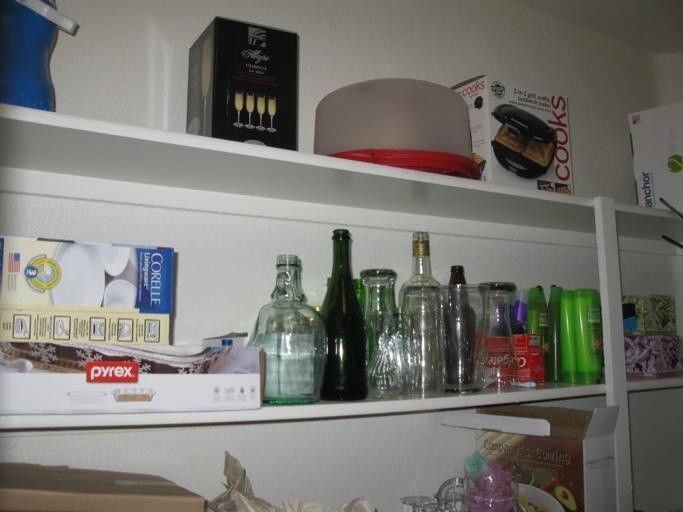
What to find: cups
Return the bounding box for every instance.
[106,245,131,276]
[103,278,138,308]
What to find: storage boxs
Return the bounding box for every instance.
[626,101,683,211]
[185,16,300,152]
[448,73,570,196]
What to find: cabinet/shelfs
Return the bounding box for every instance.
[0,101,683,512]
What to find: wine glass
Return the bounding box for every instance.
[232,88,278,134]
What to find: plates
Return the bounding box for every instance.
[50,242,106,308]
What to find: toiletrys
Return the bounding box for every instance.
[514,286,603,384]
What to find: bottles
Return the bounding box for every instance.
[247,229,521,408]
